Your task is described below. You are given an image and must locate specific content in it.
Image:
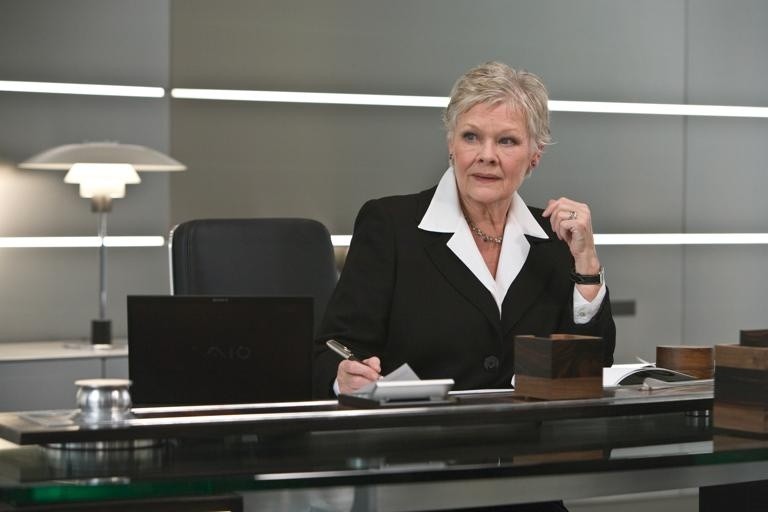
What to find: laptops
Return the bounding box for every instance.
[127,294,314,407]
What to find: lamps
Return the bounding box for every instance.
[19,142,187,413]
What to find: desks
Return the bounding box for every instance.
[1,383,768,512]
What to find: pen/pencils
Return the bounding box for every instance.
[325,339,384,381]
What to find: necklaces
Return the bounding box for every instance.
[463,209,502,245]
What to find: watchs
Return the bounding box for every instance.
[570,266,606,285]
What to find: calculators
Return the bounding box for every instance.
[338,378,456,408]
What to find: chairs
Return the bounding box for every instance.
[169,218,338,339]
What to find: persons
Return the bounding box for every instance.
[315,64,617,512]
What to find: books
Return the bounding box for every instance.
[602,362,694,388]
